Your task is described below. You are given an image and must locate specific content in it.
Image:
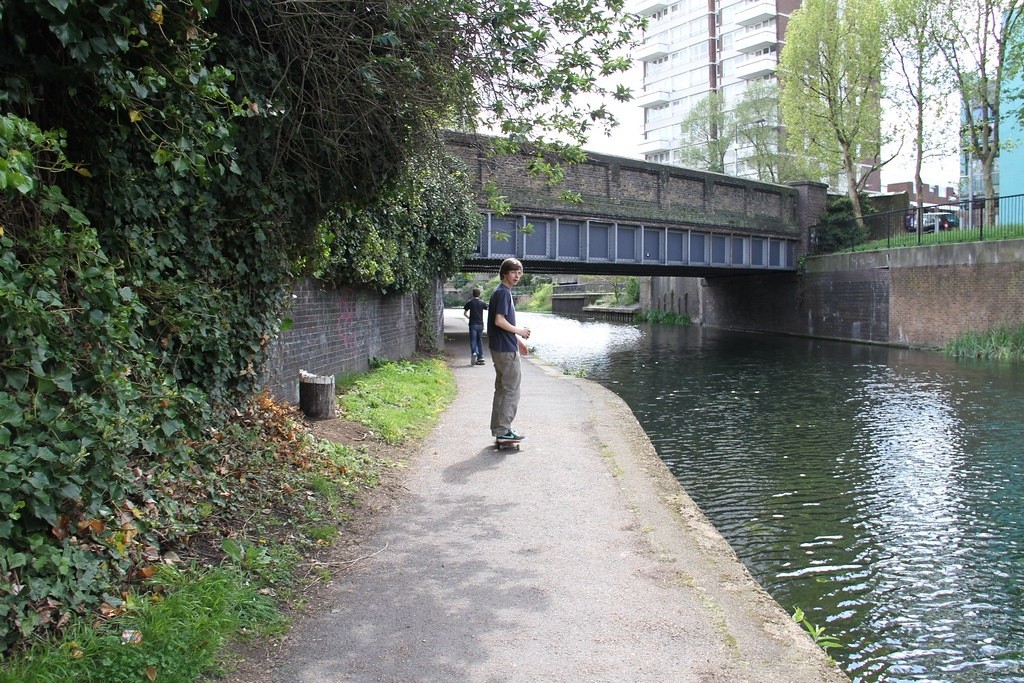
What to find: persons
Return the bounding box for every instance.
[487,258,530,443]
[464,288,490,365]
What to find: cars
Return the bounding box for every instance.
[828,186,962,232]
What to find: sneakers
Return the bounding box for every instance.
[496,430,525,443]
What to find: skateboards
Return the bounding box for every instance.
[476,360,485,365]
[496,439,521,451]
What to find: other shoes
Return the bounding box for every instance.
[471,352,477,364]
[477,356,482,361]
